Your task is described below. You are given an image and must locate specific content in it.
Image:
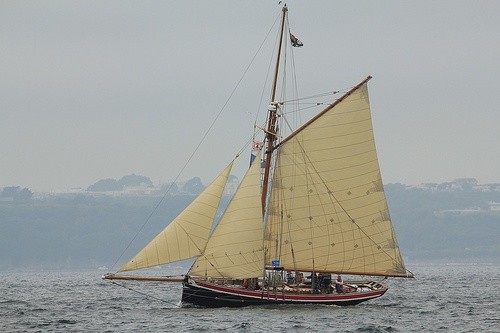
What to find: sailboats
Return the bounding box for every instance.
[99,0,413,309]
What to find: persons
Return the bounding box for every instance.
[243,268,345,294]
